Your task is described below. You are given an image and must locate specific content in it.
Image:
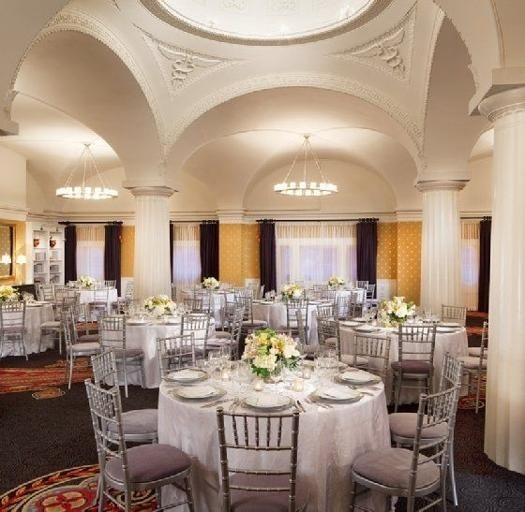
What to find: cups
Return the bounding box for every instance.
[252,377,268,391]
[301,366,314,379]
[293,379,307,392]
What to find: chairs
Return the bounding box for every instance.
[316,317,369,368]
[166,313,210,374]
[386,351,464,505]
[0,299,29,361]
[233,296,268,335]
[351,332,391,394]
[90,347,158,445]
[99,314,144,398]
[206,309,244,360]
[285,296,309,345]
[82,378,195,512]
[457,321,488,414]
[89,281,109,324]
[469,346,486,357]
[349,382,461,512]
[215,406,299,512]
[174,275,251,310]
[155,332,197,377]
[391,323,437,412]
[441,304,467,327]
[38,296,78,355]
[62,322,100,390]
[310,276,378,317]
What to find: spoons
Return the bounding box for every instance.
[305,397,320,411]
[228,396,239,411]
[349,384,374,396]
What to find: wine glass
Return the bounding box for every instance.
[425,305,433,330]
[236,363,251,394]
[207,351,219,383]
[269,364,283,394]
[220,346,233,379]
[218,353,226,380]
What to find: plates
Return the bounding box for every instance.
[166,368,208,383]
[339,319,359,327]
[305,361,350,366]
[393,325,420,336]
[189,314,209,320]
[352,316,370,323]
[316,386,363,401]
[35,299,51,304]
[438,321,462,326]
[173,385,224,401]
[430,325,454,334]
[308,301,320,304]
[261,302,274,305]
[338,370,380,384]
[25,302,44,307]
[0,304,18,309]
[353,327,381,333]
[241,394,296,410]
[157,317,180,325]
[126,318,150,325]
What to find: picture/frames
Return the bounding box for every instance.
[0,221,16,279]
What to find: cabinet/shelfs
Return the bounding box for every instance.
[27,221,65,282]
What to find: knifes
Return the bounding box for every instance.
[297,399,306,413]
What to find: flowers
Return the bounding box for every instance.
[368,296,415,325]
[0,284,18,304]
[240,329,302,377]
[144,293,174,317]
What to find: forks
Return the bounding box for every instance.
[231,401,240,414]
[200,397,231,410]
[309,396,328,410]
[312,395,334,408]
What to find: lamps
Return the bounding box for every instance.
[272,138,338,198]
[54,146,118,200]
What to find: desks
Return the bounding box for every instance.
[158,366,390,512]
[253,298,311,328]
[59,285,117,321]
[338,318,469,402]
